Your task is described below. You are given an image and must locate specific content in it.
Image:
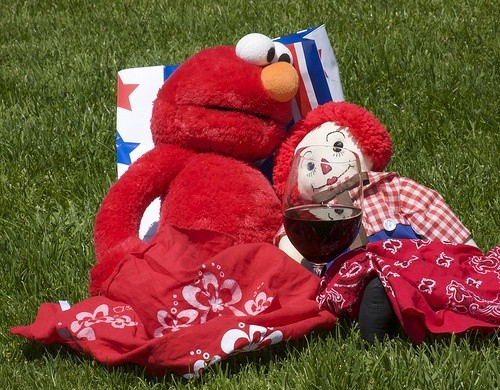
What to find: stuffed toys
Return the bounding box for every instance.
[86,28,301,296]
[270,100,486,349]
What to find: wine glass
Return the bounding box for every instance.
[281,145,364,278]
